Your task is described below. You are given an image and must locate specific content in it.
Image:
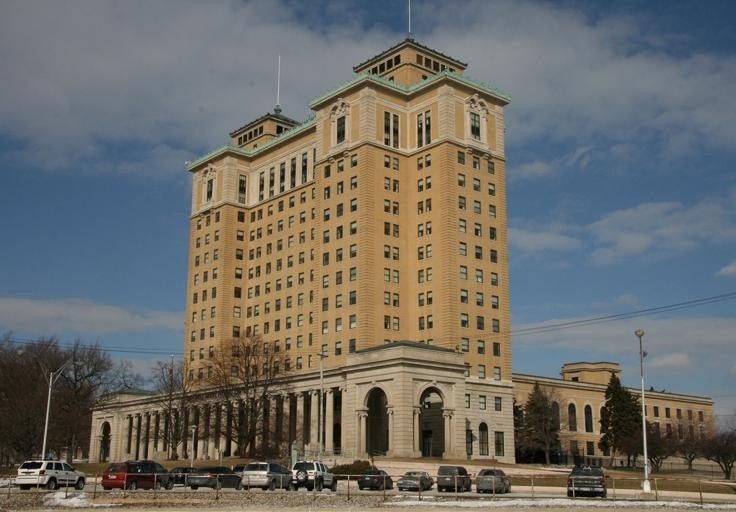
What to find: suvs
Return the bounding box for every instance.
[241,461,293,491]
[233,464,246,476]
[291,459,337,492]
[101,459,174,491]
[17,460,87,490]
[437,465,472,493]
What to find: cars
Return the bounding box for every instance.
[396,471,434,491]
[358,469,393,490]
[475,468,510,494]
[169,466,200,484]
[564,464,608,499]
[189,465,242,491]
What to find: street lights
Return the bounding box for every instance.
[634,327,652,492]
[41,355,83,459]
[163,354,175,460]
[188,424,198,467]
